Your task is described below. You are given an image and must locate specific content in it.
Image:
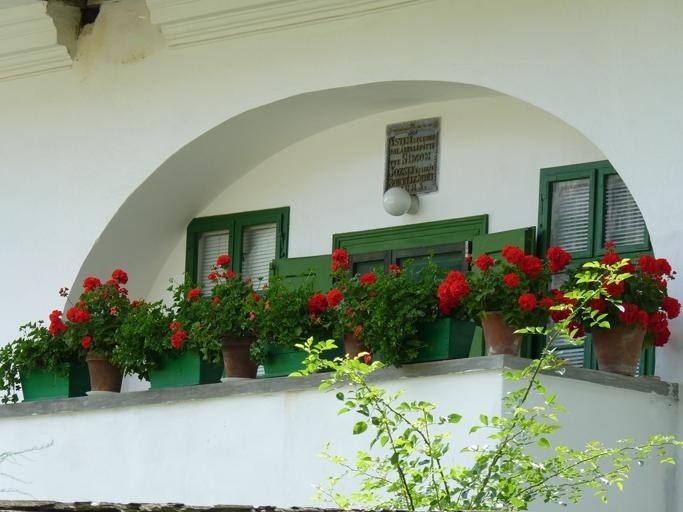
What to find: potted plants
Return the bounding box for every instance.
[110,300,207,390]
[0,312,87,405]
[248,267,344,378]
[358,248,477,362]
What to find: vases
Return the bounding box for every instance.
[335,325,365,369]
[592,323,646,381]
[211,327,255,384]
[474,304,524,357]
[84,348,124,393]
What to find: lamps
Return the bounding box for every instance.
[382,187,422,215]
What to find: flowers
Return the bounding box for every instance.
[553,242,679,345]
[441,242,582,339]
[307,248,438,362]
[160,253,267,354]
[46,267,170,361]
